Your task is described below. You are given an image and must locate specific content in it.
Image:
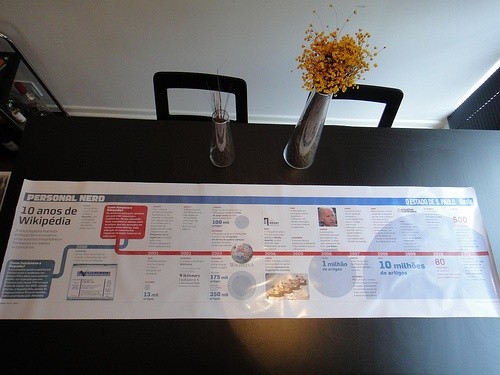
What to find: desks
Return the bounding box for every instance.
[0,115,500,375]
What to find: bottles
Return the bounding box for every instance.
[0,80,53,152]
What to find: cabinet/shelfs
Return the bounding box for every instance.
[0,33,68,130]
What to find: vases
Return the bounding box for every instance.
[209,109,235,167]
[283,88,333,169]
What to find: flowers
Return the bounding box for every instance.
[289,3,388,93]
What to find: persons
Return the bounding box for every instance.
[318,207,337,227]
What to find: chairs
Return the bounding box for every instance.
[153,72,248,122]
[308,84,403,128]
